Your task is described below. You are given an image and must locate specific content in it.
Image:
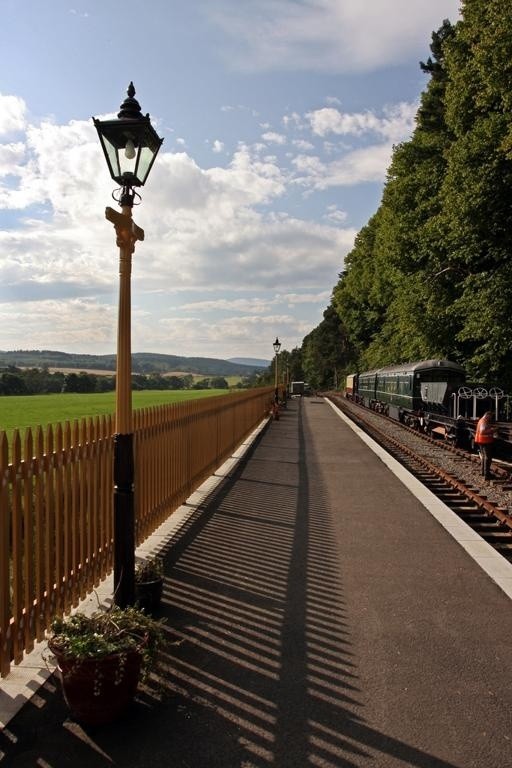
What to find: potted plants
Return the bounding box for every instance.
[135,559,163,615]
[48,606,183,727]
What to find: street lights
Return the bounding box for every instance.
[91,81,171,608]
[285,356,291,389]
[273,335,282,422]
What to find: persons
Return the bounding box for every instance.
[475,410,497,480]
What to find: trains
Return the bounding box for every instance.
[345,359,468,438]
